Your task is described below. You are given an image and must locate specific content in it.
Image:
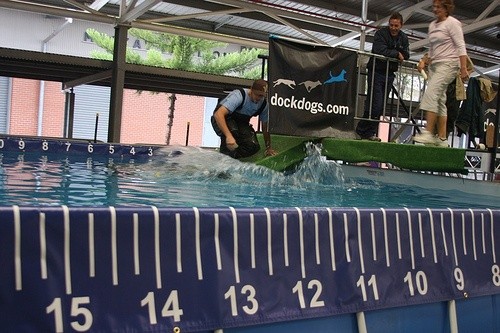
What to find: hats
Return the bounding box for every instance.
[252,80,268,97]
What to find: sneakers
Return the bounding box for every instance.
[435,137,449,146]
[411,129,436,144]
[352,129,381,142]
